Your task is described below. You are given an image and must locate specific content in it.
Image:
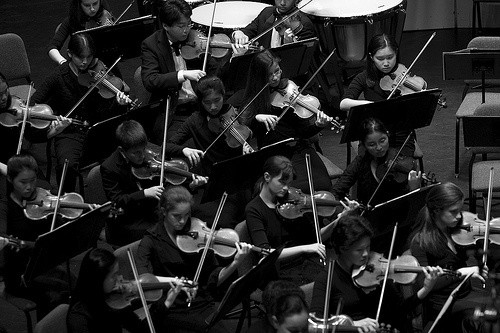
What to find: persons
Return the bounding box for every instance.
[410,182,500,333]
[0,75,72,178]
[309,214,443,333]
[65,249,184,333]
[165,76,250,193]
[43,0,130,94]
[241,49,332,193]
[341,32,426,173]
[330,117,423,260]
[133,184,252,312]
[26,34,130,196]
[0,154,103,319]
[230,0,319,84]
[246,156,360,283]
[102,119,207,247]
[247,281,357,333]
[143,0,248,127]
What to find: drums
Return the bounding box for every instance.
[294,0,408,123]
[190,1,275,39]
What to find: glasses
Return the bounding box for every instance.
[269,68,283,80]
[170,21,194,30]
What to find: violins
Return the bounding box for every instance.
[93,9,119,26]
[177,216,273,258]
[374,149,439,185]
[78,58,143,111]
[268,78,345,133]
[450,209,500,246]
[274,12,303,43]
[209,103,257,155]
[131,150,208,185]
[278,186,365,220]
[306,313,396,333]
[351,250,461,289]
[0,95,89,129]
[106,271,195,309]
[181,29,260,60]
[379,63,448,110]
[22,186,102,221]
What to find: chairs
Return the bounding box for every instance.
[455,36,500,214]
[0,20,423,333]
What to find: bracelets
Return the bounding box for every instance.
[59,59,65,64]
